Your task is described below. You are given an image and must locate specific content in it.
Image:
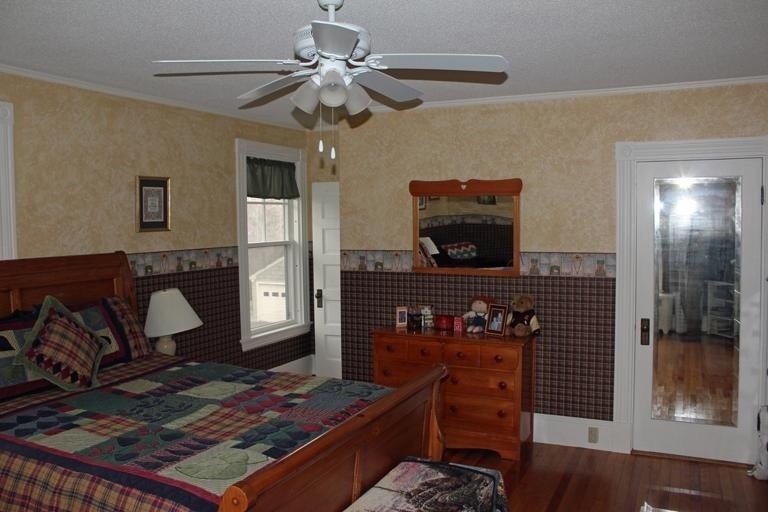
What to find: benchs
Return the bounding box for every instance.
[344,455,507,512]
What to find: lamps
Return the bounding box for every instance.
[289,59,372,159]
[144,287,205,356]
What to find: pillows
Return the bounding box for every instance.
[0,295,152,401]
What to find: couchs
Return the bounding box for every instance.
[420,213,513,268]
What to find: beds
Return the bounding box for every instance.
[0,249,449,511]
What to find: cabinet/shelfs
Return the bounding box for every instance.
[368,326,536,460]
[706,279,733,338]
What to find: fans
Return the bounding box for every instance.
[150,0,509,104]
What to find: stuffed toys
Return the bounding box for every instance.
[504,295,541,337]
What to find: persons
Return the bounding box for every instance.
[462,295,494,333]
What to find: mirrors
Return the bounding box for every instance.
[653,174,742,428]
[408,178,523,277]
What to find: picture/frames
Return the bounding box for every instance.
[476,196,496,205]
[419,196,427,210]
[428,196,440,200]
[485,304,508,337]
[135,175,171,232]
[395,306,408,327]
